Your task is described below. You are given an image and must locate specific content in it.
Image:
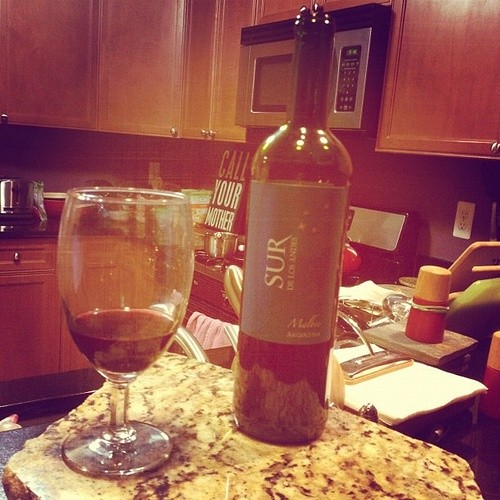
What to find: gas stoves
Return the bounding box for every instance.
[187,202,418,317]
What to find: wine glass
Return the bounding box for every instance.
[55,186,195,476]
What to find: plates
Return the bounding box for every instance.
[398,277,417,288]
[377,283,414,297]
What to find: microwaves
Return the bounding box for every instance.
[234,2,391,140]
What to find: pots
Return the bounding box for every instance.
[204,231,239,259]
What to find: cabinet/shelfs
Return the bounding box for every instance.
[374,0,500,159]
[184,0,257,144]
[254,0,393,26]
[154,254,242,370]
[95,0,186,139]
[0,237,138,423]
[0,0,100,132]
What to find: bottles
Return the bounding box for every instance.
[478,331,500,420]
[404,263,452,344]
[233,4,354,445]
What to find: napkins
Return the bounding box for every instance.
[336,281,404,316]
[327,344,489,426]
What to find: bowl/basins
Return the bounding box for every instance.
[43,200,65,218]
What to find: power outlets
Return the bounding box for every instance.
[453,200,476,240]
